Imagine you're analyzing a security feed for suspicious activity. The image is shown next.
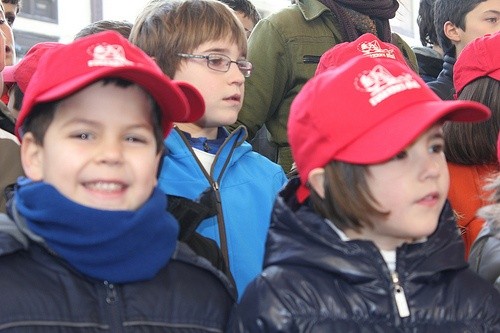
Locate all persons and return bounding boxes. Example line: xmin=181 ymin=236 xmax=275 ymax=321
xmin=127 ymin=0 xmax=289 ymax=304
xmin=224 ymin=55 xmax=500 ymax=333
xmin=218 ymin=0 xmax=281 ymax=164
xmin=0 ymin=0 xmax=134 ymax=212
xmin=409 ymin=0 xmax=444 ymax=84
xmin=425 ymin=0 xmax=500 ymax=101
xmin=446 ymin=28 xmax=500 ymax=260
xmin=0 ymin=30 xmax=241 ymax=333
xmin=226 ymin=0 xmax=419 ymax=170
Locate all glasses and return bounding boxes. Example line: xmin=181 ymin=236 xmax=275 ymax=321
xmin=176 ymin=53 xmax=252 ymax=78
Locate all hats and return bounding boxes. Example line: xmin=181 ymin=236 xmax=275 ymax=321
xmin=315 ymin=33 xmax=409 ymax=73
xmin=288 ymin=52 xmax=491 ymax=204
xmin=452 ymin=30 xmax=500 ymax=101
xmin=17 ymin=29 xmax=188 ymax=146
xmin=169 ymin=80 xmax=208 ymax=124
xmin=1 ymin=42 xmax=60 ymax=94
